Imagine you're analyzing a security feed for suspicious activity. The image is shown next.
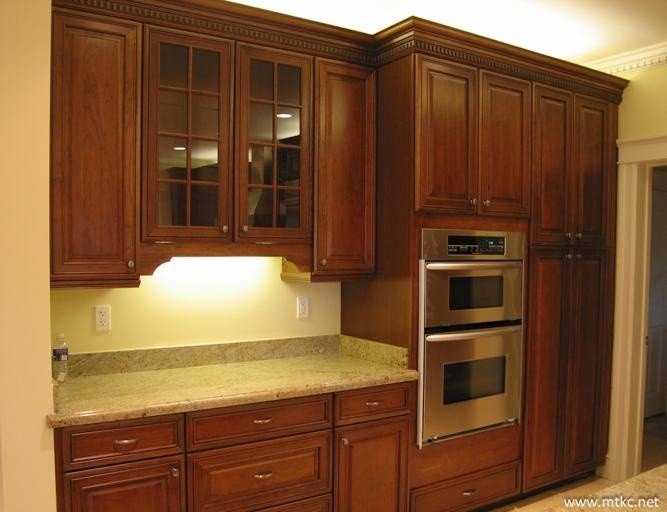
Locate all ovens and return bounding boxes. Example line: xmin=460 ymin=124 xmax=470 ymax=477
xmin=422 ymin=260 xmax=523 ymax=443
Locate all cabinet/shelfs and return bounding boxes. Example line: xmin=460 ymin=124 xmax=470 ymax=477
xmin=280 ymin=51 xmax=379 ymax=282
xmin=379 ymin=55 xmax=534 ymax=221
xmin=49 ymin=11 xmax=141 ymax=280
xmin=410 ymin=458 xmax=521 ymax=511
xmin=61 ymin=412 xmax=184 ymax=511
xmin=332 ymin=383 xmax=412 ymax=512
xmin=184 ymin=392 xmax=331 ymax=511
xmin=142 ymin=21 xmax=314 ymax=243
xmin=518 ymin=79 xmax=608 ymax=491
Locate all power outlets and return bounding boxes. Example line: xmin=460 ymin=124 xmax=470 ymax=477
xmin=94 ymin=305 xmax=111 ymax=332
xmin=296 ymin=296 xmax=309 ymax=319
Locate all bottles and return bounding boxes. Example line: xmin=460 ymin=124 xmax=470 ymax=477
xmin=52 ymin=331 xmax=70 ymax=386
